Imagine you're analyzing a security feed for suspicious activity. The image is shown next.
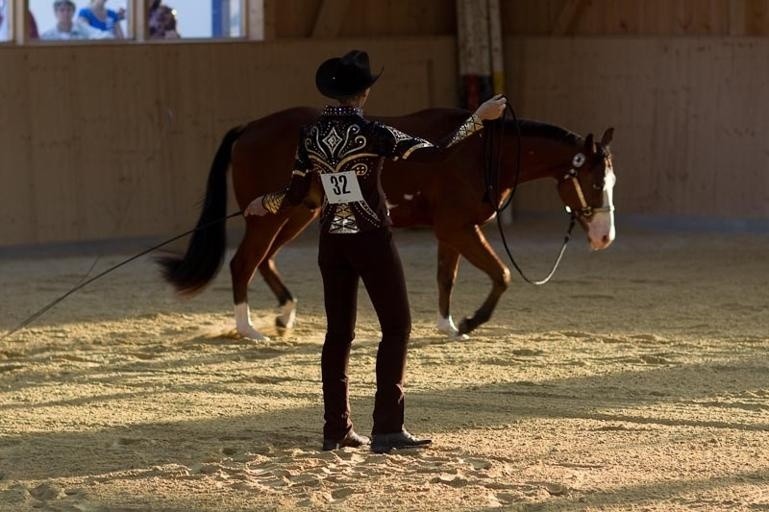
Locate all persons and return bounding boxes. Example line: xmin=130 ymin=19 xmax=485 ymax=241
xmin=243 ymin=50 xmax=507 ymax=453
xmin=75 ymin=0 xmax=126 ymax=40
xmin=0 ymin=1 xmax=39 ymax=43
xmin=39 ymin=1 xmax=87 ymax=42
xmin=146 ymin=1 xmax=180 ymax=41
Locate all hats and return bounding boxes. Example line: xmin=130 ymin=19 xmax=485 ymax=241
xmin=314 ymin=48 xmax=386 ymax=100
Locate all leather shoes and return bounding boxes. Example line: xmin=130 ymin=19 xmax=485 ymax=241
xmin=322 ymin=428 xmax=372 ymax=447
xmin=370 ymin=428 xmax=432 ymax=452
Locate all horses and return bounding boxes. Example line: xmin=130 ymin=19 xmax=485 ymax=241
xmin=156 ymin=106 xmax=617 ymax=341
